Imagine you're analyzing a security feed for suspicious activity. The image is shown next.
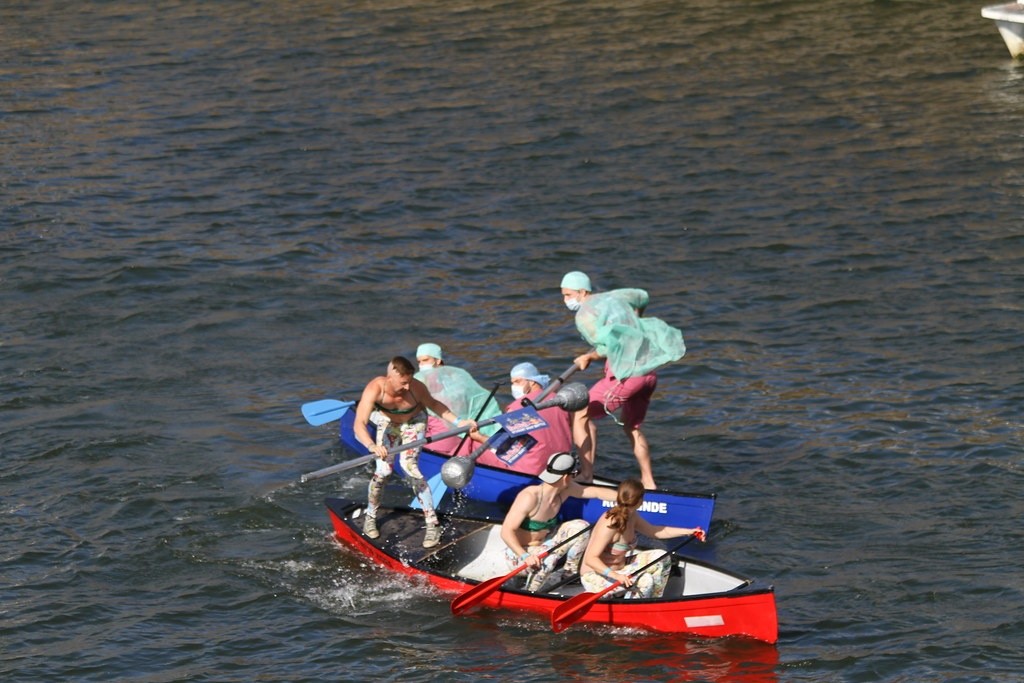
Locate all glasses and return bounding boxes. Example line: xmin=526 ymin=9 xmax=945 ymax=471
xmin=570 ymin=468 xmax=578 ymax=478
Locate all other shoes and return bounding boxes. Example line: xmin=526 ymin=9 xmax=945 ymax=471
xmin=560 ymin=574 xmax=582 ymax=587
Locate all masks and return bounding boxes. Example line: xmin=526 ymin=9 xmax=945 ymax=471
xmin=564 ymin=294 xmax=583 ymax=312
xmin=511 ymin=382 xmax=531 ymax=400
xmin=419 ymin=362 xmax=436 ymax=372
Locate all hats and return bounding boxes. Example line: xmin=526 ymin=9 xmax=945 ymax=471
xmin=538 ymin=452 xmax=576 ymax=483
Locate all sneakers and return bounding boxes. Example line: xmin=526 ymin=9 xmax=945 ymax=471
xmin=362 ymin=514 xmax=379 ymax=539
xmin=422 ymin=522 xmax=444 ymax=548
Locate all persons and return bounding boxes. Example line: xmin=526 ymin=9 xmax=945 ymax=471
xmin=560 ymin=271 xmax=686 ymax=489
xmin=501 ymin=451 xmax=618 ymax=594
xmin=413 ymin=343 xmax=503 ymax=456
xmin=579 ymin=478 xmax=705 ymax=599
xmin=353 ymin=356 xmax=477 ymax=548
xmin=449 ymin=362 xmax=574 ymax=479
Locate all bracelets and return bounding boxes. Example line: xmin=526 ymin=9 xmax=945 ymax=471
xmin=603 ymin=567 xmax=611 ymax=576
xmin=451 ymin=417 xmax=460 ymax=426
xmin=520 ymin=552 xmax=530 ymax=561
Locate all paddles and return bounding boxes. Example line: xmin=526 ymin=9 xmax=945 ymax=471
xmin=301 ymin=398 xmax=360 ymax=427
xmin=550 ymin=525 xmax=704 ymax=633
xmin=410 ymin=380 xmax=508 ymax=510
xmin=451 ymin=522 xmax=597 ymax=618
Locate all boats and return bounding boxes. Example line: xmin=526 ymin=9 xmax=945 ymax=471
xmin=323 ymin=496 xmax=779 ymax=645
xmin=982 ymin=0 xmax=1024 ymax=59
xmin=338 ymin=405 xmax=715 ymax=532
